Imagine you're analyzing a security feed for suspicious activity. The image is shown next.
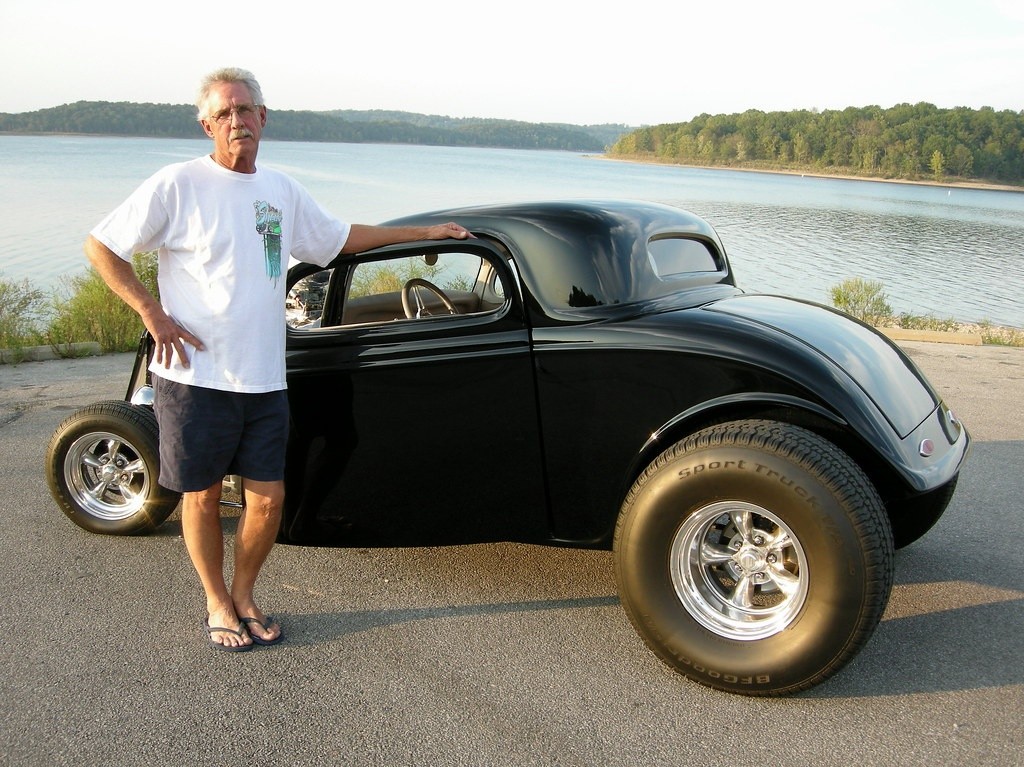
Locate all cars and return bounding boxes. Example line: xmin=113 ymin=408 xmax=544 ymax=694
xmin=47 ymin=201 xmax=970 ymax=697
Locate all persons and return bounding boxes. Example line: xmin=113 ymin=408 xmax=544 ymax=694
xmin=83 ymin=69 xmax=481 ymax=652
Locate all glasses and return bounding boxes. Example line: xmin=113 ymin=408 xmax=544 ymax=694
xmin=209 ymin=102 xmax=261 ymax=124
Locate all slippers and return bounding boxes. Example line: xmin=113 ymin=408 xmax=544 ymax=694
xmin=243 ymin=616 xmax=284 ymax=645
xmin=204 ymin=618 xmax=254 ymax=652
